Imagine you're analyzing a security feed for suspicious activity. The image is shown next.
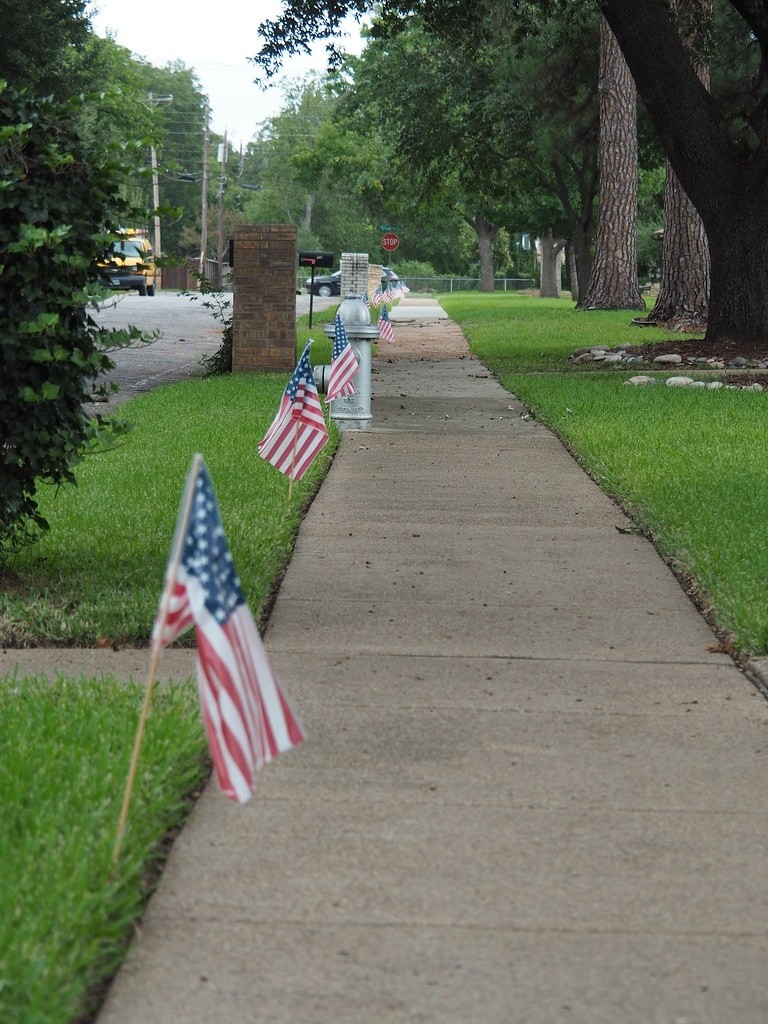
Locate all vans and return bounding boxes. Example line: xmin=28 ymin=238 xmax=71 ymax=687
xmin=93 ymin=228 xmax=160 ymax=297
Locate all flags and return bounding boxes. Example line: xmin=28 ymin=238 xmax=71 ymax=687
xmin=377 ymin=306 xmax=394 ymax=344
xmin=258 ymin=339 xmax=328 ymax=482
xmin=324 ymin=313 xmax=359 ymax=403
xmin=382 ymin=281 xmax=410 ymax=304
xmin=373 ymin=284 xmax=382 ymax=308
xmin=362 ymin=289 xmax=370 ymax=313
xmin=152 ymin=456 xmax=304 ymax=804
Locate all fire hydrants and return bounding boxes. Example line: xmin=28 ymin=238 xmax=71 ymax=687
xmin=312 ymin=292 xmax=378 ymax=434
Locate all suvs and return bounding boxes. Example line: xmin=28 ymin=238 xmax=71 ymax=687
xmin=305 ymin=265 xmax=400 ymax=296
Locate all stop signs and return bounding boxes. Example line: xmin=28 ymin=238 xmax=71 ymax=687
xmin=380 ymin=233 xmax=400 ymax=252
